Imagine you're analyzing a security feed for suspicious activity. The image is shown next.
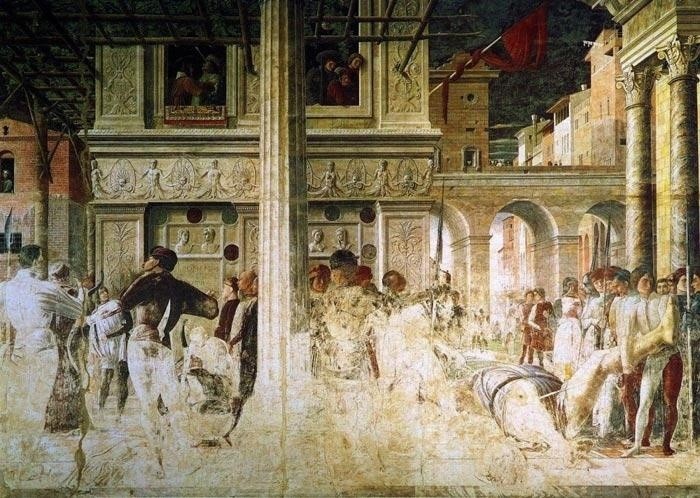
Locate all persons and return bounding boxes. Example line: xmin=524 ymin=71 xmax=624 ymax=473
xmin=228 ymin=178 xmax=261 ymax=190
xmin=90 ymin=160 xmax=109 ymax=200
xmin=310 ymin=160 xmax=353 ymax=197
xmin=419 ymin=157 xmax=434 ymax=196
xmin=134 ymin=158 xmax=171 ymax=200
xmin=110 ymin=177 xmax=133 ymax=194
xmin=342 ymin=174 xmax=373 ymax=188
xmin=392 ymin=174 xmax=423 ymax=194
xmin=164 ymin=177 xmax=201 ymax=192
xmin=194 ymin=159 xmax=234 ymax=199
xmin=0 ymin=169 xmax=13 ymax=193
xmin=367 ymin=158 xmax=397 ymax=198
xmin=166 ymin=48 xmax=363 ymax=110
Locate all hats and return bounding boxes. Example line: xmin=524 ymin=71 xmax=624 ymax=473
xmin=355 ymin=266 xmax=373 ymax=281
xmin=150 ymin=245 xmax=178 ymax=267
xmin=308 ymin=263 xmax=331 ymax=280
xmin=382 ymin=270 xmax=408 ymax=292
xmin=562 ymin=267 xmax=693 ymax=292
xmin=329 ymin=249 xmax=360 ymax=269
xmin=48 ymin=260 xmax=71 ymax=282
